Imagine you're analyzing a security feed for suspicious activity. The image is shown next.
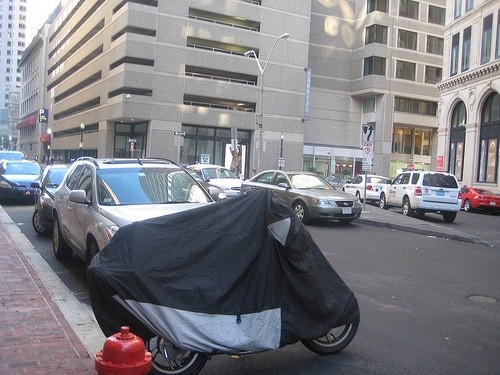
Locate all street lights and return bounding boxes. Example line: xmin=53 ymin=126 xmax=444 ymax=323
xmin=244 ymin=33 xmax=290 ymax=175
xmin=79 ymin=121 xmax=87 ymax=159
xmin=278 ymin=133 xmax=284 ymax=172
xmin=46 ymin=128 xmax=53 ymax=164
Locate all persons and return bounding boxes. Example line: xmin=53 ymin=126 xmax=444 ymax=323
xmin=25 ymin=154 xmax=54 ymax=166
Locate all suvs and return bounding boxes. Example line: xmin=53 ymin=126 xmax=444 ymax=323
xmin=51 ymin=155 xmax=219 ymax=274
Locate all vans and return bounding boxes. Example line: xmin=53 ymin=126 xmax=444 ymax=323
xmin=380 ymin=171 xmax=462 ymax=222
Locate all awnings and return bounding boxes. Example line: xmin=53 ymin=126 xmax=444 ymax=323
xmin=16 ymin=115 xmax=36 ymax=130
xmin=40 ymin=134 xmax=50 ymax=142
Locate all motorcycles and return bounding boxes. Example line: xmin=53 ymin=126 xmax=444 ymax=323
xmin=84 ymin=184 xmax=359 ymax=375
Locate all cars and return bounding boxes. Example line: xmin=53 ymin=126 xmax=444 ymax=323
xmin=0 ymin=159 xmax=43 ymax=206
xmin=458 ymin=186 xmax=500 ymax=212
xmin=242 ymin=170 xmax=363 ymax=224
xmin=341 ymin=174 xmax=391 ymax=203
xmin=30 ymin=164 xmax=75 ymax=235
xmin=1 ymin=151 xmax=26 ymax=162
xmin=187 ymin=164 xmax=244 ymax=201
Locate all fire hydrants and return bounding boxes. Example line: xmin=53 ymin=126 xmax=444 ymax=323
xmin=94 ymin=325 xmax=155 ymax=375
